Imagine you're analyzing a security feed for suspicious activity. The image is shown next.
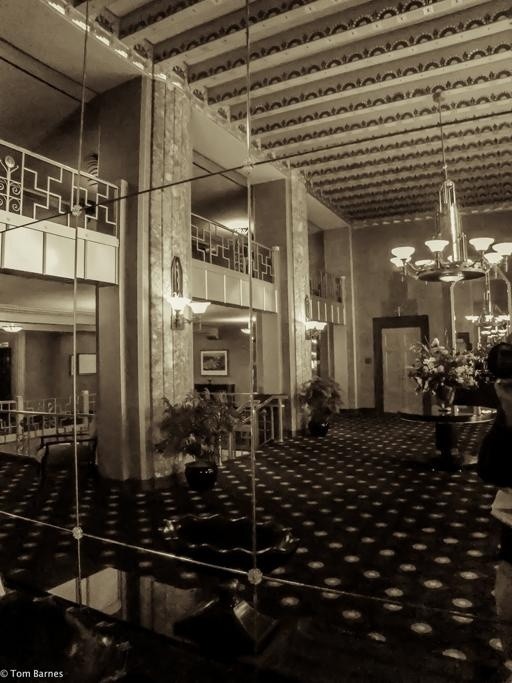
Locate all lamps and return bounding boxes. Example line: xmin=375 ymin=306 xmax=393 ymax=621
xmin=167 ymin=297 xmax=211 ymax=330
xmin=2 ymin=326 xmax=23 ymax=333
xmin=233 ymin=228 xmax=248 ymax=236
xmin=305 ymin=321 xmax=327 ymax=340
xmin=389 ymin=91 xmax=512 ymax=283
xmin=464 ymin=272 xmax=510 ymax=342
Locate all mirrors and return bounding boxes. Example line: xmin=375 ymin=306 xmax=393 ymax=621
xmin=449 ymin=264 xmax=511 ymax=355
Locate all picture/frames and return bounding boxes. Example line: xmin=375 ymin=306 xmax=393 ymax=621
xmin=77 ymin=352 xmax=97 ymax=375
xmin=200 ymin=349 xmax=230 ymax=377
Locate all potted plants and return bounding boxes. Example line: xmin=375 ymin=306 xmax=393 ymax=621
xmin=154 ymin=388 xmax=246 ymax=490
xmin=297 ymin=375 xmax=344 ymax=437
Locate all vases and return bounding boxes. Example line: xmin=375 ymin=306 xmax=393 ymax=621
xmin=430 ymin=384 xmax=456 ymax=412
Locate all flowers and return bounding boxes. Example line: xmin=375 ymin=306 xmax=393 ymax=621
xmin=405 ymin=328 xmax=483 ymax=395
